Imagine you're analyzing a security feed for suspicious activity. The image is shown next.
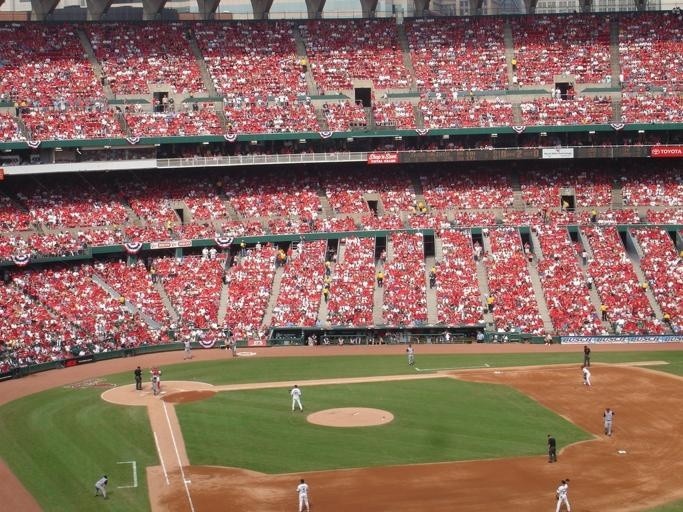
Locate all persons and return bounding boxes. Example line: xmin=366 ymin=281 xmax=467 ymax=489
xmin=0 ymin=11 xmax=682 ymax=375
xmin=135 ymin=367 xmax=142 ymax=390
xmin=290 ymin=385 xmax=303 ymax=412
xmin=94 ymin=475 xmax=109 ymax=499
xmin=602 ymin=408 xmax=616 ymax=436
xmin=296 ymin=478 xmax=310 ymax=512
xmin=546 ymin=434 xmax=557 ymax=463
xmin=555 ymin=478 xmax=571 ymax=512
xmin=149 ymin=367 xmax=162 ymax=391
xmin=580 ymin=365 xmax=593 ymax=391
